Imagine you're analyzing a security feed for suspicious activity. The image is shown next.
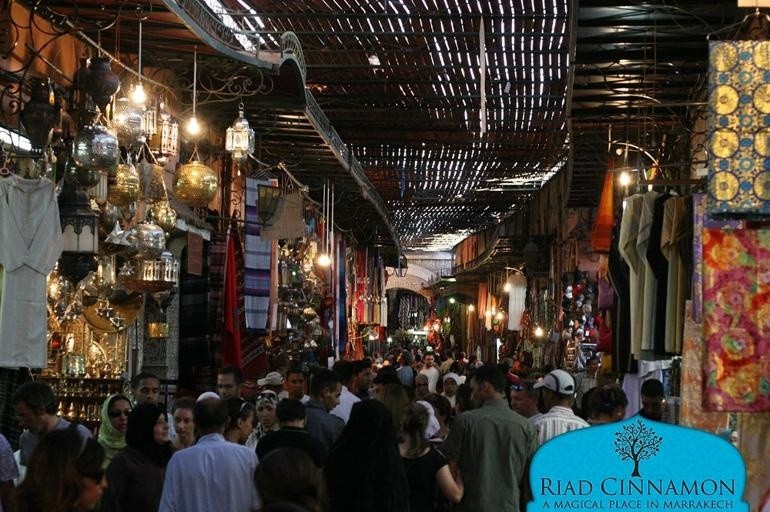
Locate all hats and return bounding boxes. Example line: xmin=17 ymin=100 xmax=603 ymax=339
xmin=257 ymin=372 xmax=285 ymax=386
xmin=533 ymin=369 xmax=575 ymax=395
xmin=416 ymin=400 xmax=441 ymax=439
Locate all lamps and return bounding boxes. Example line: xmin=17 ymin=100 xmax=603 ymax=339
xmin=185 ymin=45 xmax=203 ymax=135
xmin=128 ymin=18 xmax=148 ymax=105
xmin=225 ymin=97 xmax=257 ymax=178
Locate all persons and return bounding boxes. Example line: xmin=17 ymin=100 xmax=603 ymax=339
xmin=0 ymin=317 xmax=663 ymax=512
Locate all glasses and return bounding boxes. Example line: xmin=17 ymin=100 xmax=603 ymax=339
xmin=109 ymin=408 xmax=132 ymax=417
